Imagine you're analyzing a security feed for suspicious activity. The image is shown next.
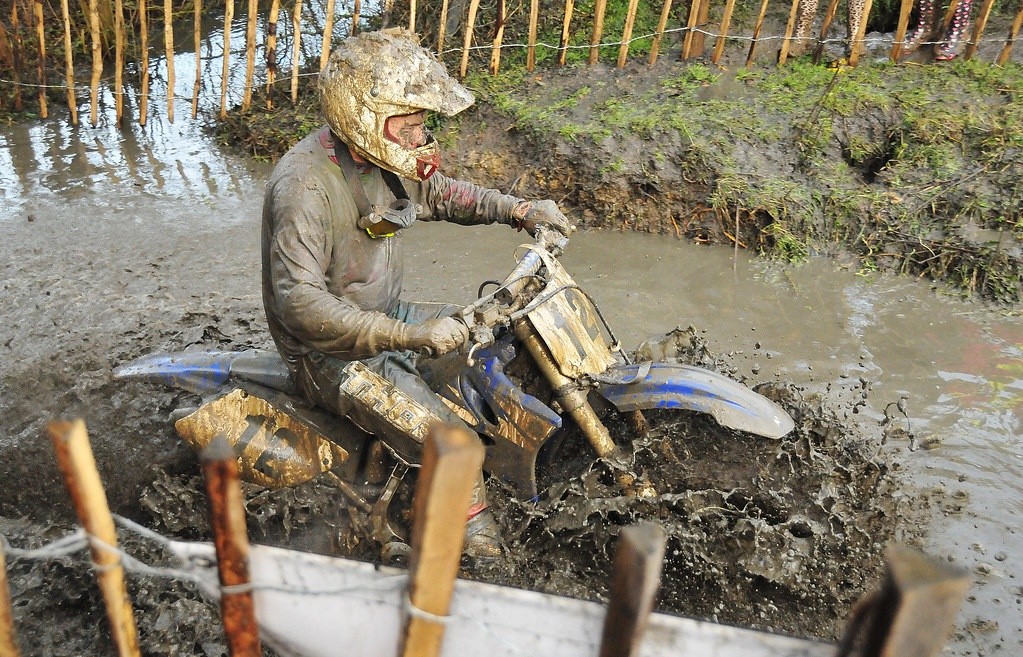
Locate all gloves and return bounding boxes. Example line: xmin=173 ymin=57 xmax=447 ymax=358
xmin=398 ymin=315 xmax=470 ymax=358
xmin=513 ymin=197 xmax=572 ymax=238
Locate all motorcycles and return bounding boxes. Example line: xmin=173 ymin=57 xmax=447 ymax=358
xmin=108 ymin=225 xmax=848 ymax=625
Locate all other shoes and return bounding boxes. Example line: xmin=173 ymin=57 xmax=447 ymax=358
xmin=461 ymin=509 xmax=502 ymax=560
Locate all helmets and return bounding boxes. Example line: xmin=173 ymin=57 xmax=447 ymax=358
xmin=316 ymin=31 xmax=475 ymax=183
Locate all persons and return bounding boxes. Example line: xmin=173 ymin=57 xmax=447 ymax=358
xmin=263 ymin=29 xmax=576 ymax=558
